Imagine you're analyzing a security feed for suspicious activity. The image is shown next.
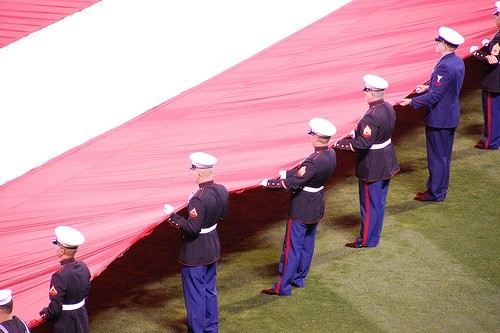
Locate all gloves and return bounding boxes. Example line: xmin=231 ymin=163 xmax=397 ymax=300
xmin=481 ymin=39 xmax=489 ymax=46
xmin=164 ymin=204 xmax=174 ymax=214
xmin=259 ymin=178 xmax=268 ymax=188
xmin=469 ymin=46 xmax=479 ymax=53
xmin=350 ymin=130 xmax=355 ymax=139
xmin=279 ymin=170 xmax=287 ymax=180
xmin=188 ymin=192 xmax=195 ymax=202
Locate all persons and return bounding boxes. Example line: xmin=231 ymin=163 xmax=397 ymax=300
xmin=0 ymin=289 xmax=32 ymax=333
xmin=469 ymin=0 xmax=500 ymax=150
xmin=398 ymin=26 xmax=466 ymax=202
xmin=163 ymin=152 xmax=230 ymax=333
xmin=333 ymin=74 xmax=401 ymax=248
xmin=258 ymin=118 xmax=337 ymax=296
xmin=38 ymin=226 xmax=92 ymax=333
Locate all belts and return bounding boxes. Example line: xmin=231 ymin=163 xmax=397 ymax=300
xmin=299 ymin=185 xmax=324 ymax=193
xmin=199 ymin=223 xmax=218 ymax=234
xmin=369 ymin=138 xmax=391 ymax=149
xmin=61 ymin=298 xmax=86 ymax=311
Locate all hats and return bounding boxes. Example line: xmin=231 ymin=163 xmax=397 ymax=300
xmin=306 ymin=117 xmax=336 ymax=138
xmin=0 ymin=289 xmax=12 ymax=306
xmin=493 ymin=1 xmax=500 ymax=15
xmin=361 ymin=74 xmax=389 ymax=91
xmin=52 ymin=226 xmax=85 ymax=249
xmin=188 ymin=152 xmax=217 ymax=170
xmin=435 ymin=26 xmax=466 ymax=47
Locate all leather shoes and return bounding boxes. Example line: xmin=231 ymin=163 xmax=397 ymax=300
xmin=414 ymin=191 xmax=428 ymax=201
xmin=261 ymin=288 xmax=279 ymax=295
xmin=344 ymin=240 xmax=368 ymax=248
xmin=474 ymin=139 xmax=498 ymax=150
